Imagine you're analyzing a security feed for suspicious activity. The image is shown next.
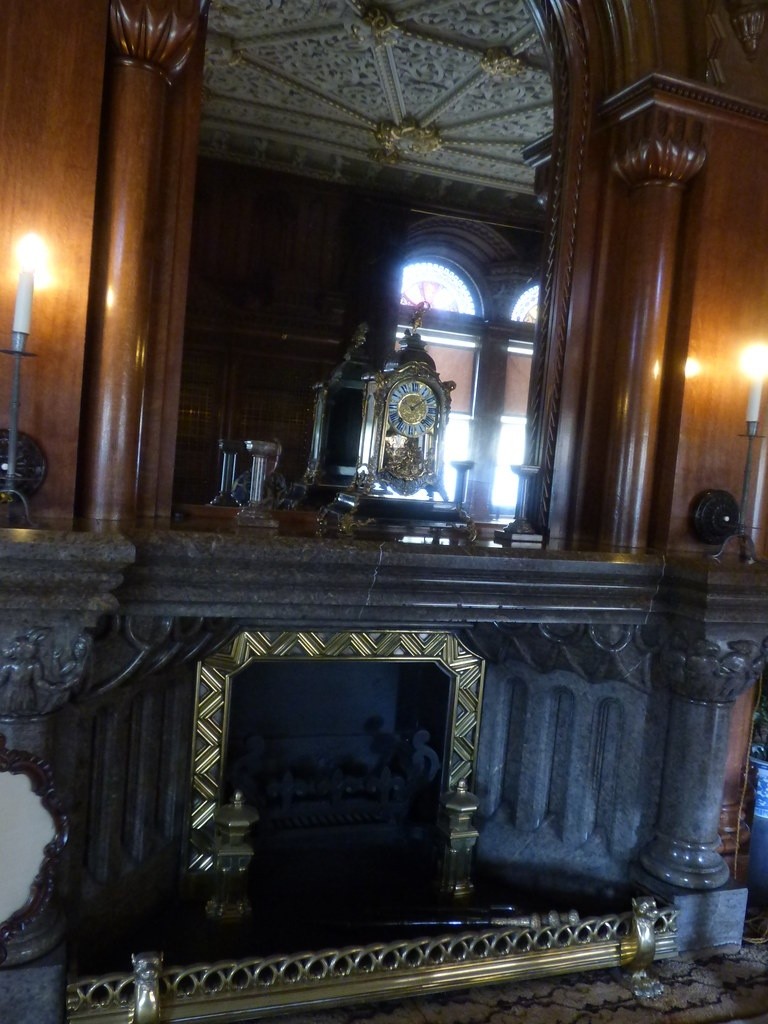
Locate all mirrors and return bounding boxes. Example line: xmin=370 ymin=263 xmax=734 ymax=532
xmin=172 ymin=0 xmax=565 ymax=531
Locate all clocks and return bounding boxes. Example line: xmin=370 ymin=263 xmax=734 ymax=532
xmin=276 ymin=322 xmax=379 ymax=511
xmin=315 ymin=332 xmax=478 ymax=545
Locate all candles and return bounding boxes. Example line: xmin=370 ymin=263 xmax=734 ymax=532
xmin=740 ymin=340 xmax=768 ymax=422
xmin=13 ymin=233 xmax=37 ymax=334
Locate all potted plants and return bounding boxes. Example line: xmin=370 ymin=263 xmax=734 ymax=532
xmin=744 ymin=695 xmax=768 ymax=818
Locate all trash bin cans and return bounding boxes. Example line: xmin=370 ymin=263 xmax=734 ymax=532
xmin=747 ymin=742 xmax=768 ymax=907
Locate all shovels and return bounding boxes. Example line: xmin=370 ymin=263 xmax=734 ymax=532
xmin=309 ymin=880 xmax=517 ymax=929
